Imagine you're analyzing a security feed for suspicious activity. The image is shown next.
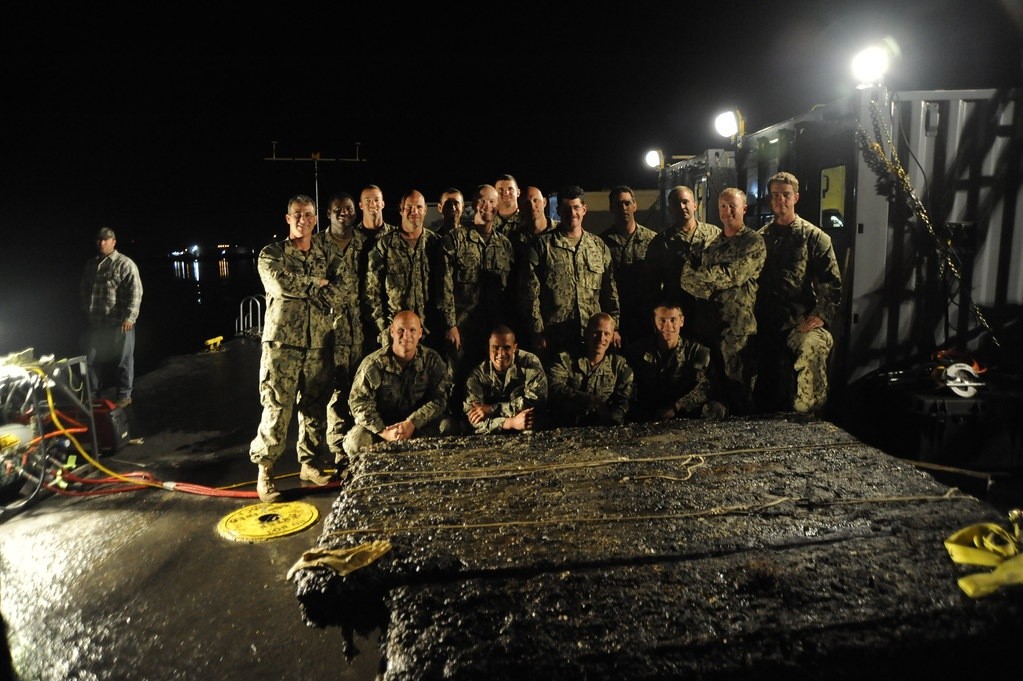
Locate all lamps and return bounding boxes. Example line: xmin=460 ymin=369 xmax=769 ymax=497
xmin=645 ymin=149 xmax=664 ymax=171
xmin=714 ymin=109 xmax=744 ymax=145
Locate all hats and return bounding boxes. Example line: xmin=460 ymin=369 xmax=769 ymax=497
xmin=97 ymin=227 xmax=115 ymax=239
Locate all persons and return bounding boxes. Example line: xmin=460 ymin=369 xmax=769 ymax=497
xmin=463 ymin=324 xmax=549 ymax=436
xmin=756 ymin=171 xmax=843 ymax=415
xmin=596 ymin=185 xmax=658 ymax=313
xmin=630 ymin=302 xmax=727 ymax=422
xmin=256 ymin=194 xmax=336 ymax=505
xmin=680 ymin=186 xmax=767 ymax=403
xmin=355 ymin=171 xmax=621 ymax=368
xmin=547 ymin=312 xmax=634 ymax=426
xmin=631 ymin=185 xmax=723 ymax=300
xmin=313 ymin=194 xmax=370 ymax=463
xmin=83 ymin=227 xmax=143 ymax=411
xmin=341 ymin=310 xmax=454 ymax=458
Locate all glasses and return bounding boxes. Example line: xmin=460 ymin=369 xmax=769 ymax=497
xmin=288 ymin=213 xmax=316 ymax=218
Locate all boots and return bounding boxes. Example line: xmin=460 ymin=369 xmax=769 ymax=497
xmin=257 ymin=463 xmax=282 ymax=502
xmin=301 ymin=463 xmax=333 ymax=486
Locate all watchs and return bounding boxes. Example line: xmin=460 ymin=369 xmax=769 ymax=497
xmin=491 ymin=403 xmax=502 ymax=416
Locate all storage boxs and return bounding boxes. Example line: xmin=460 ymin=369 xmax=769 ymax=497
xmin=901 ymin=371 xmax=1023 ymax=468
